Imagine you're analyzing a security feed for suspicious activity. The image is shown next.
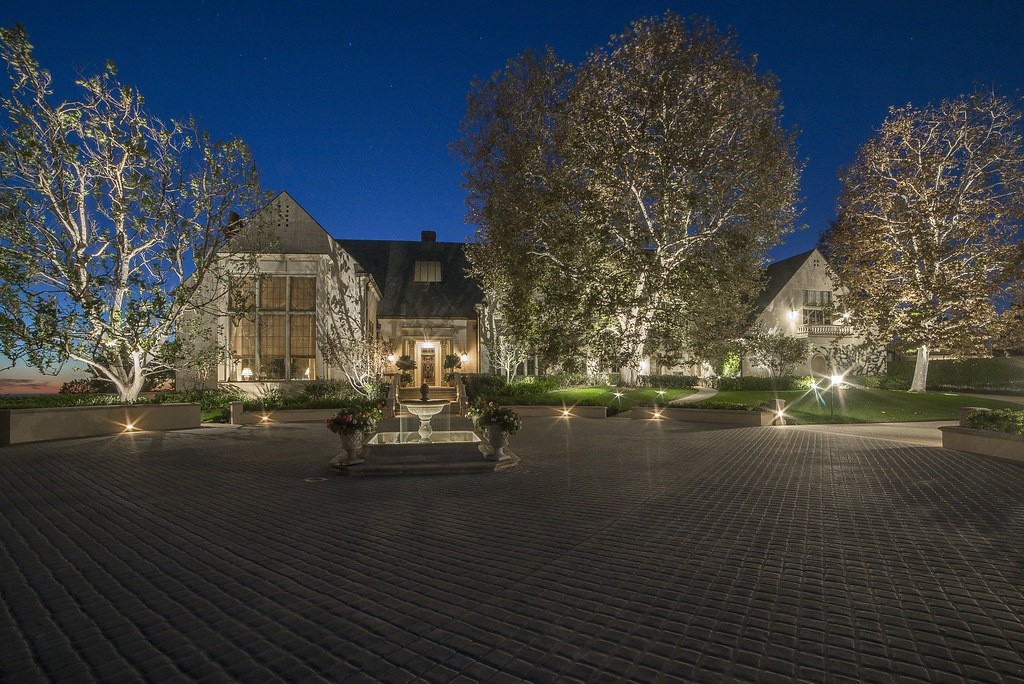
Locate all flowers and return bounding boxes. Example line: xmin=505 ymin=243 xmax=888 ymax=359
xmin=359 ymin=404 xmax=384 ymax=424
xmin=325 ymin=405 xmax=377 ymax=435
xmin=476 ymin=401 xmax=523 ymax=435
xmin=465 ymin=397 xmax=496 ymax=420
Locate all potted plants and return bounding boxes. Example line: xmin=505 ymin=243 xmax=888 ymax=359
xmin=396 ymin=354 xmax=418 ymax=387
xmin=442 ymin=353 xmax=462 ymax=387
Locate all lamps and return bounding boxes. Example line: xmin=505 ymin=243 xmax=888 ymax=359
xmin=241 ymin=368 xmax=253 ymax=381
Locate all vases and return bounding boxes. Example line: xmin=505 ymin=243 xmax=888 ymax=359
xmin=482 ymin=429 xmax=511 ymax=461
xmin=337 ymin=431 xmax=364 ymax=465
